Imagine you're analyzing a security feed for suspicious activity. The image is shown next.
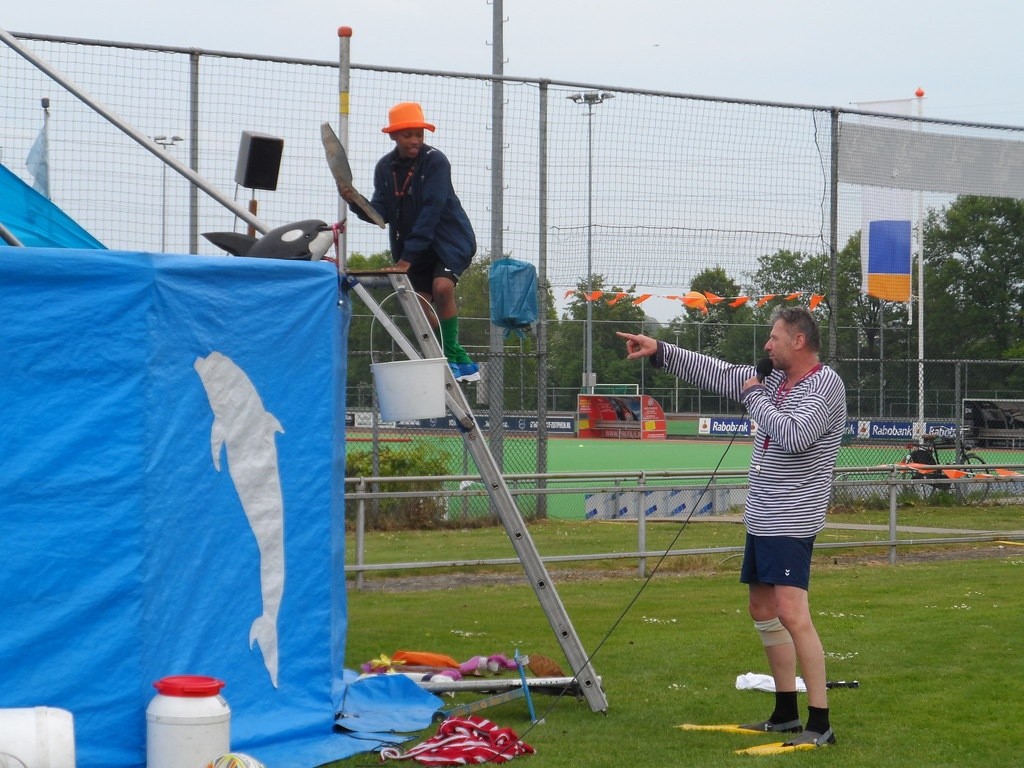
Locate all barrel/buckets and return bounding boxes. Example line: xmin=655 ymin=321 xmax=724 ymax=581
xmin=369 ymin=290 xmax=446 ymax=423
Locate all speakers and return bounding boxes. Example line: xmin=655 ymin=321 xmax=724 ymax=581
xmin=235 ymin=130 xmax=284 ymax=192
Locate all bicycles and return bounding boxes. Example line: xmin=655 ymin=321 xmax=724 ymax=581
xmin=902 ymin=426 xmax=991 ymax=508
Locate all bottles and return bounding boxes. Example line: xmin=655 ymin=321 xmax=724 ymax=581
xmin=146 ymin=677 xmax=231 ymax=768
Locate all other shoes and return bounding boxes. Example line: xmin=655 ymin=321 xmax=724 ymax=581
xmin=448 ymin=361 xmax=481 ymax=383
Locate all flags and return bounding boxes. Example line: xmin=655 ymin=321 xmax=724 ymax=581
xmin=860 ymin=103 xmax=912 ymax=303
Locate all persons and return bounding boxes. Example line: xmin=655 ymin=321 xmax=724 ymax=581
xmin=338 ymin=100 xmax=479 ymax=383
xmin=615 ymin=307 xmax=846 ymax=748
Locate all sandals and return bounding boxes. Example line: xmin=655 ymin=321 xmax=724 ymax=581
xmin=782 ymin=726 xmax=834 ymax=747
xmin=739 ymin=719 xmax=802 ymax=733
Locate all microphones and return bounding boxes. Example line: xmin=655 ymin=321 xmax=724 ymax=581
xmin=756 ymin=358 xmax=773 ymax=384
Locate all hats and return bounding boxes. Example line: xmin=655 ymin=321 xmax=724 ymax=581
xmin=382 ymin=102 xmax=435 ymax=131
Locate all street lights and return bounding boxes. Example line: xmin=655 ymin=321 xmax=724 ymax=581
xmin=566 ymin=90 xmax=616 ymax=393
xmin=149 ymin=133 xmax=184 ymax=253
